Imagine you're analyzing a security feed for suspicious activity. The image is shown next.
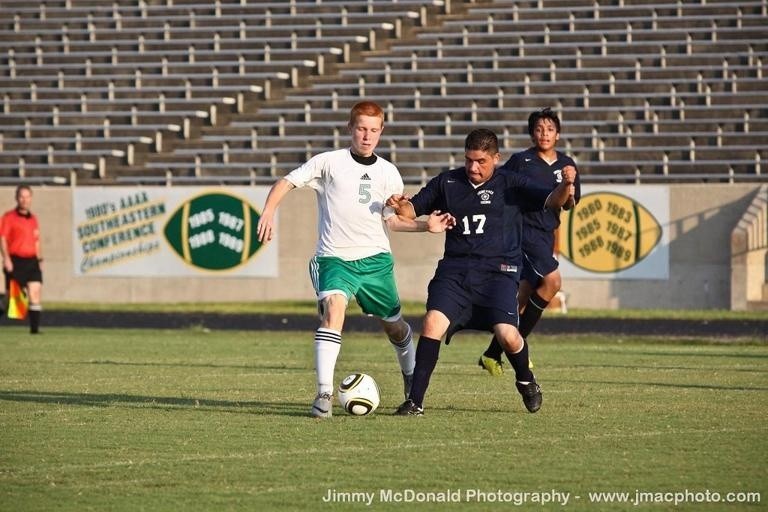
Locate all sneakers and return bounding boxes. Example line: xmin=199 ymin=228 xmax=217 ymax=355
xmin=515 ymin=371 xmax=542 ymax=413
xmin=402 ymin=370 xmax=414 ymax=400
xmin=478 ymin=354 xmax=505 ymax=376
xmin=392 ymin=398 xmax=424 ymax=416
xmin=311 ymin=391 xmax=334 ymax=417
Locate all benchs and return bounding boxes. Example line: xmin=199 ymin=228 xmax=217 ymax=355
xmin=0 ymin=0 xmax=768 ymax=186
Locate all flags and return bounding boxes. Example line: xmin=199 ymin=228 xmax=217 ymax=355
xmin=8 ymin=277 xmax=29 ymax=321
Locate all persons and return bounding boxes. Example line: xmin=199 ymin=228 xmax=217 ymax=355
xmin=0 ymin=183 xmax=45 ymax=333
xmin=387 ymin=128 xmax=577 ymax=417
xmin=256 ymin=101 xmax=456 ymax=419
xmin=477 ymin=108 xmax=581 ymax=379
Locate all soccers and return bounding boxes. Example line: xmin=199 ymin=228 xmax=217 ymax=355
xmin=339 ymin=373 xmax=380 ymax=415
